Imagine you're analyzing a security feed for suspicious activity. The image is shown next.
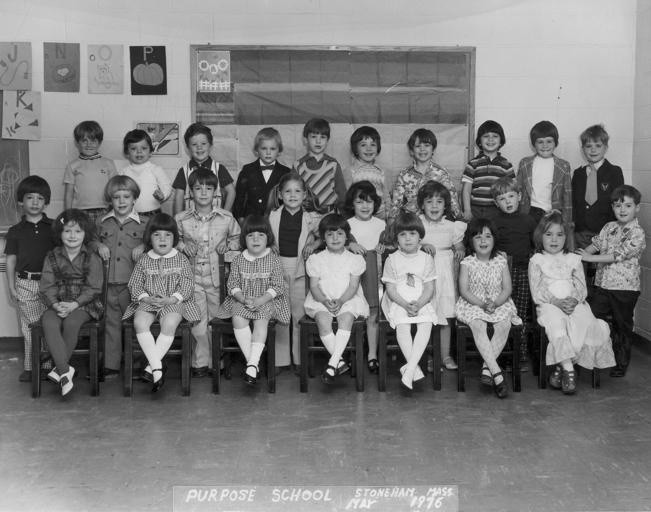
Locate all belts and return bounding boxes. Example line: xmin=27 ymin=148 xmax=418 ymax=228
xmin=18 ymin=271 xmax=42 ymax=280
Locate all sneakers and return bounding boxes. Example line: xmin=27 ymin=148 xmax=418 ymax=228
xmin=562 ymin=370 xmax=577 ymax=395
xmin=549 ymin=364 xmax=562 ymax=389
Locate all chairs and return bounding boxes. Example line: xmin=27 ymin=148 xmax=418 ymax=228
xmin=208 ymin=250 xmax=279 ymax=393
xmin=298 ymin=249 xmax=365 ymax=392
xmin=530 ymin=253 xmax=601 ymax=389
xmin=122 ymin=248 xmax=194 ymax=397
xmin=28 ymin=249 xmax=110 ymax=397
xmin=455 ymin=255 xmax=524 ymax=392
xmin=375 ymin=247 xmax=441 ymax=392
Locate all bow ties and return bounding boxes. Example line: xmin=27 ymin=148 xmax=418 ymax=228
xmin=258 ymin=164 xmax=275 ymax=171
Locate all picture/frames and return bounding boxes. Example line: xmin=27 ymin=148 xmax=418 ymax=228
xmin=133 ymin=120 xmax=184 ymax=158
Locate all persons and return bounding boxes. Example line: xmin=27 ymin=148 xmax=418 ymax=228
xmin=129 ymin=212 xmax=200 ymax=393
xmin=236 ymin=127 xmax=293 ymax=224
xmin=4 ymin=175 xmax=58 ymax=382
xmin=41 ymin=209 xmax=104 ymax=395
xmin=456 ymin=219 xmax=524 ymax=399
xmin=383 ymin=213 xmax=448 ymax=391
xmin=293 ymin=118 xmax=350 ymax=225
xmin=343 ymin=181 xmax=389 ymax=377
xmin=415 ymin=182 xmax=467 ymax=374
xmin=485 ymin=179 xmax=541 ymax=373
xmin=349 ymin=126 xmax=393 ymax=224
xmin=174 ymin=168 xmax=245 ymax=377
xmin=460 ymin=121 xmax=517 ymax=221
xmin=571 ymin=125 xmax=625 ymax=254
xmin=265 ymin=173 xmax=326 ymax=377
xmin=516 ymin=121 xmax=574 ymax=248
xmin=88 ymin=175 xmax=154 ymax=379
xmin=63 ymin=121 xmax=120 ymax=231
xmin=306 ymin=213 xmax=371 ymax=383
xmin=217 ymin=214 xmax=293 ymax=385
xmin=529 ymin=213 xmax=618 ymax=392
xmin=116 ymin=130 xmax=173 ymax=218
xmin=576 ymin=185 xmax=648 ymax=377
xmin=171 ymin=123 xmax=237 ymax=216
xmin=391 ymin=128 xmax=464 ymax=232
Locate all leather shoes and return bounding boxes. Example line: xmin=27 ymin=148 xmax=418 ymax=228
xmin=322 ymin=363 xmax=337 ymax=385
xmin=191 ymin=367 xmax=208 ymax=377
xmin=490 ymin=368 xmax=509 ymax=398
xmin=87 ymin=369 xmax=120 ymax=380
xmin=337 ymin=358 xmax=351 ymax=376
xmin=208 ymin=368 xmax=222 ymax=377
xmin=48 ymin=367 xmax=59 ymax=383
xmin=399 ymin=364 xmax=414 ymax=393
xmin=443 ymin=357 xmax=459 ymax=370
xmin=413 ymin=366 xmax=426 ymax=384
xmin=240 ymin=363 xmax=261 ymax=385
xmin=609 ymin=365 xmax=626 ymax=377
xmin=151 ymin=360 xmax=168 ymax=394
xmin=141 ymin=364 xmax=154 ymax=382
xmin=40 ymin=368 xmax=49 ymax=380
xmin=59 ymin=364 xmax=78 ymax=396
xmin=479 ymin=366 xmax=493 ymax=386
xmin=428 ymin=359 xmax=443 ymax=373
xmin=367 ymin=359 xmax=380 ymax=374
xmin=19 ymin=370 xmax=32 ymax=382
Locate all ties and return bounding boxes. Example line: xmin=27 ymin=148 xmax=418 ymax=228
xmin=584 ymin=161 xmax=597 ymax=205
